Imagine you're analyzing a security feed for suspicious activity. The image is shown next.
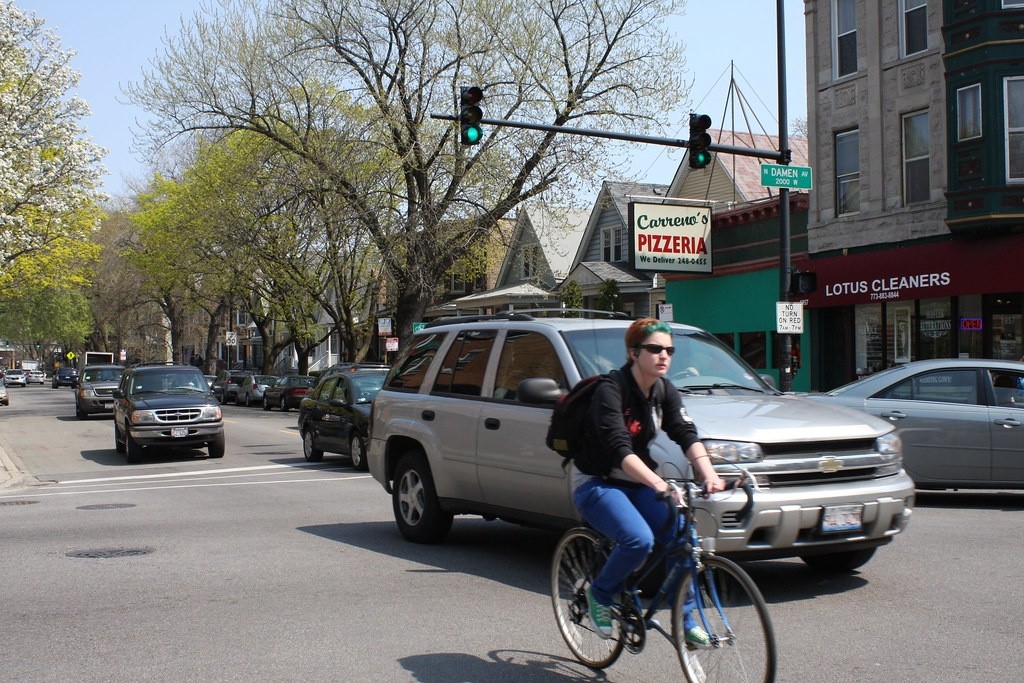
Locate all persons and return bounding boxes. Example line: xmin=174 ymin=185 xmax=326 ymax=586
xmin=994 ymin=376 xmax=1014 ymax=404
xmin=344 ymin=386 xmax=361 ymax=404
xmin=569 ymin=318 xmax=728 ymax=649
xmin=993 ymin=329 xmax=1002 ymax=353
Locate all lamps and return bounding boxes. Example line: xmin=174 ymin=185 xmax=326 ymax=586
xmin=842 ymin=247 xmax=849 ymax=256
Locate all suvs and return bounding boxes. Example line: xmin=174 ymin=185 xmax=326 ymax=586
xmin=209 ymin=370 xmax=258 ymax=405
xmin=73 ymin=362 xmax=127 ymax=421
xmin=366 ymin=306 xmax=917 ymax=596
xmin=51 ymin=366 xmax=77 ymax=389
xmin=112 ymin=360 xmax=227 ymax=463
xmin=306 ymin=361 xmax=391 ymax=395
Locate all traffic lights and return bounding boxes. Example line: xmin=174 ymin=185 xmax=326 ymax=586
xmin=6 ymin=341 xmax=10 ymax=348
xmin=459 ymin=85 xmax=484 ymax=145
xmin=792 ymin=271 xmax=818 ymax=295
xmin=37 ymin=342 xmax=40 ymax=347
xmin=688 ymin=113 xmax=712 ymax=169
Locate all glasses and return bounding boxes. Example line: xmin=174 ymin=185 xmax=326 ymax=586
xmin=634 ymin=344 xmax=676 ymax=357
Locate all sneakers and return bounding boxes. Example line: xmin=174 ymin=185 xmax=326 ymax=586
xmin=670 ymin=625 xmax=722 ymax=650
xmin=586 ymin=586 xmax=612 ymax=639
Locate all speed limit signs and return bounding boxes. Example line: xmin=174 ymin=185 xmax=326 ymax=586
xmin=225 ymin=331 xmax=237 ymax=346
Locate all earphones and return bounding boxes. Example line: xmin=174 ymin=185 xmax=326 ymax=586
xmin=634 ymin=350 xmax=639 ymax=357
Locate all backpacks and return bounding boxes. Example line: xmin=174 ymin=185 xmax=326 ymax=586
xmin=546 ymin=369 xmax=665 ymax=460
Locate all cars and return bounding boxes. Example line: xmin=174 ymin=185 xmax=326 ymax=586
xmin=235 ymin=374 xmax=280 ymax=407
xmin=297 ymin=369 xmax=390 ymax=470
xmin=203 ymin=374 xmax=218 ymax=388
xmin=262 ymin=376 xmax=318 ymax=412
xmin=786 ymin=357 xmax=1024 ymax=491
xmin=0 ymin=360 xmax=45 ymax=406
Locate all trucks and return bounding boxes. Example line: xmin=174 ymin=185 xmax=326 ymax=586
xmin=76 ymin=352 xmax=114 ymax=384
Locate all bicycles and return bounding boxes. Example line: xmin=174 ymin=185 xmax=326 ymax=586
xmin=550 ymin=454 xmax=777 ymax=683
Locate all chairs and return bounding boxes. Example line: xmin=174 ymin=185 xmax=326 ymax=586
xmin=993 ymin=375 xmax=1018 ymax=406
